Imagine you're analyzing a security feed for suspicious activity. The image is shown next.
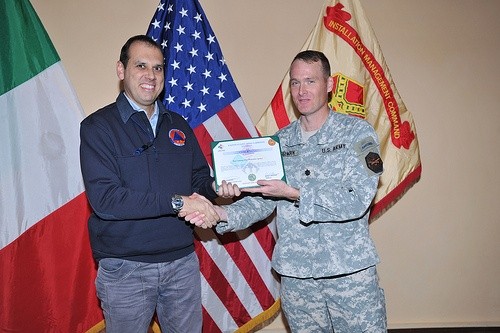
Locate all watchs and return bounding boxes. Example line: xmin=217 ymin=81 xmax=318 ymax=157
xmin=171 ymin=193 xmax=185 ymax=214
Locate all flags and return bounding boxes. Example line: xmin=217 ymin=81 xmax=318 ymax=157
xmin=256 ymin=0 xmax=423 ymax=221
xmin=1 ymin=0 xmax=106 ymax=333
xmin=146 ymin=0 xmax=283 ymax=333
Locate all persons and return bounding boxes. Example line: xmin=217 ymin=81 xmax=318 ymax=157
xmin=179 ymin=50 xmax=387 ymax=333
xmin=79 ymin=34 xmax=240 ymax=333
